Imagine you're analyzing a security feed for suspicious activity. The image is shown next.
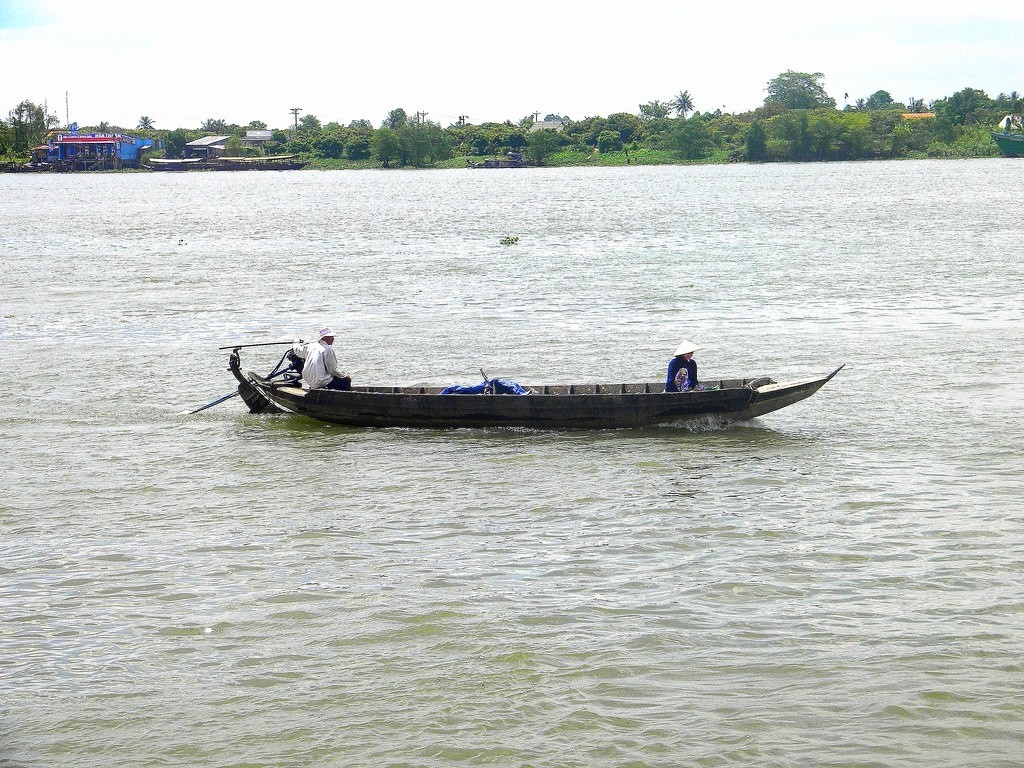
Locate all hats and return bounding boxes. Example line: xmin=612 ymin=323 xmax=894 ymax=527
xmin=673 ymin=340 xmax=705 ymax=356
xmin=318 ymin=327 xmax=338 ymax=343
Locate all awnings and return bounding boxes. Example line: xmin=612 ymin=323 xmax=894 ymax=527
xmin=30 ymin=144 xmax=59 ymax=162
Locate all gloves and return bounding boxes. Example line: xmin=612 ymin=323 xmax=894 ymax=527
xmin=293 ymin=336 xmax=300 ymax=343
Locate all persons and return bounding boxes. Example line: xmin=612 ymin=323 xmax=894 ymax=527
xmin=626 ymin=155 xmax=638 ymax=164
xmin=293 ymin=327 xmax=351 ymax=391
xmin=1006 ymin=118 xmax=1012 ymax=130
xmin=666 ymin=339 xmax=706 ymax=392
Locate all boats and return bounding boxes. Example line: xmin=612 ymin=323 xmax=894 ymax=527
xmin=989 ymin=131 xmax=1024 ymax=156
xmin=217 ymin=338 xmax=847 ymax=429
xmin=465 ymin=152 xmax=535 ymax=169
xmin=148 ymin=155 xmax=307 ymax=171
